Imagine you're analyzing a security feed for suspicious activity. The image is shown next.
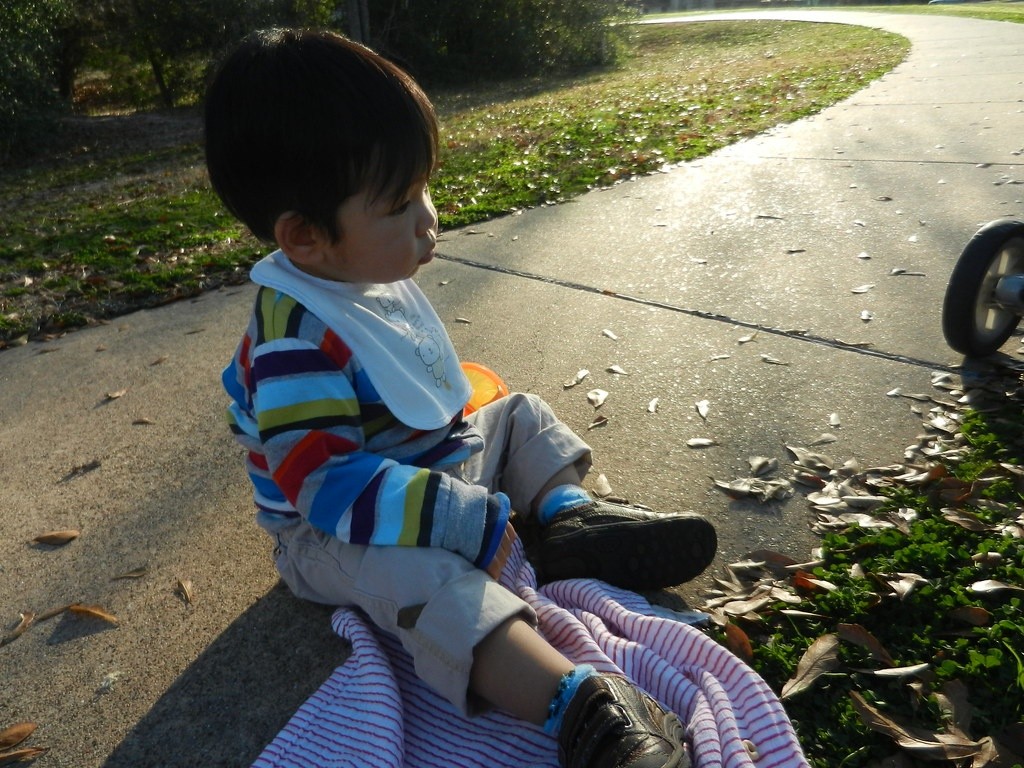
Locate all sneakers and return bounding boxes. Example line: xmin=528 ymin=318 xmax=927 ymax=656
xmin=538 ymin=495 xmax=718 ymax=592
xmin=556 ymin=676 xmax=692 ymax=768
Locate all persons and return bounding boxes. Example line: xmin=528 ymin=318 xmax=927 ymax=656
xmin=200 ymin=24 xmax=718 ymax=768
